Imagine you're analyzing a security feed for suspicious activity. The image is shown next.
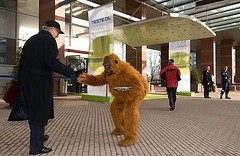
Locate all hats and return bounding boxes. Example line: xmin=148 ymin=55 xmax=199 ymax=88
xmin=45 ymin=20 xmax=65 ymax=34
xmin=168 ymin=58 xmax=174 ymax=63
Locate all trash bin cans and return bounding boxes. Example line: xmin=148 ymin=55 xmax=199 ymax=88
xmin=60 ymin=75 xmax=68 ymax=96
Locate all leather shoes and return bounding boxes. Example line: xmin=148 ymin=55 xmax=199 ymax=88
xmin=44 ymin=135 xmax=49 ymax=142
xmin=29 ymin=145 xmax=53 ymax=155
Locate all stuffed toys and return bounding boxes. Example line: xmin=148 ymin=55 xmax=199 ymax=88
xmin=76 ymin=53 xmax=147 ymax=147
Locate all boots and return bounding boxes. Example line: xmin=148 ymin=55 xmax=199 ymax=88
xmin=225 ymin=95 xmax=231 ymax=99
xmin=204 ymin=94 xmax=211 ymax=98
xmin=220 ymin=94 xmax=222 ymax=99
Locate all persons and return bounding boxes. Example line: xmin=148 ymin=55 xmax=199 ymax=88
xmin=160 ymin=59 xmax=181 ymax=110
xmin=19 ymin=20 xmax=78 ymax=155
xmin=202 ymin=66 xmax=216 ymax=98
xmin=220 ymin=65 xmax=231 ymax=99
